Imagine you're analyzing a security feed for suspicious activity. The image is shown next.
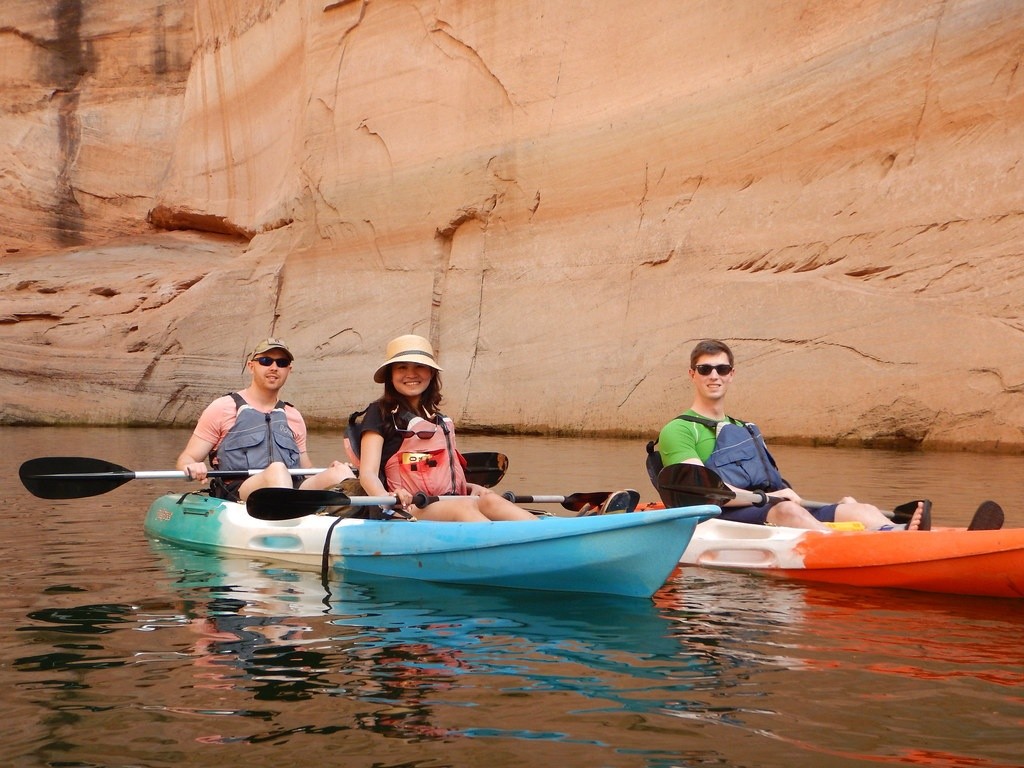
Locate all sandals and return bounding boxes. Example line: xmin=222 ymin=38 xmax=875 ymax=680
xmin=967 ymin=500 xmax=1005 ymax=530
xmin=904 ymin=498 xmax=932 ymax=531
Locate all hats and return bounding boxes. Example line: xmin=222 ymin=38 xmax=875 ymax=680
xmin=373 ymin=334 xmax=444 ymax=384
xmin=252 ymin=337 xmax=294 ymax=363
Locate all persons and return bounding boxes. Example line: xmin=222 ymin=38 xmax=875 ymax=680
xmin=172 ymin=338 xmax=363 ymax=516
xmin=358 ymin=335 xmax=642 ymax=521
xmin=659 ymin=340 xmax=1006 ymax=534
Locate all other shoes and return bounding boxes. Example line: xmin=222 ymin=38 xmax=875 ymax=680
xmin=597 ymin=488 xmax=631 ymax=515
xmin=624 ymin=489 xmax=640 ymax=512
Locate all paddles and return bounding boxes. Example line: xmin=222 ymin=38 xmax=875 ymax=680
xmin=657 ymin=463 xmax=933 ymax=524
xmin=246 ymin=485 xmax=641 ymax=521
xmin=18 ymin=449 xmax=511 ymax=501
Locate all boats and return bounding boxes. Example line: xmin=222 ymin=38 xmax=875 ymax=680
xmin=141 ymin=490 xmax=722 ymax=600
xmin=590 ymin=499 xmax=1023 ymax=601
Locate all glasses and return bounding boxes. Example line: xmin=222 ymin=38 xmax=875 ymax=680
xmin=252 ymin=356 xmax=290 ymax=369
xmin=391 ymin=406 xmax=438 ymax=440
xmin=693 ymin=363 xmax=733 ymax=376
